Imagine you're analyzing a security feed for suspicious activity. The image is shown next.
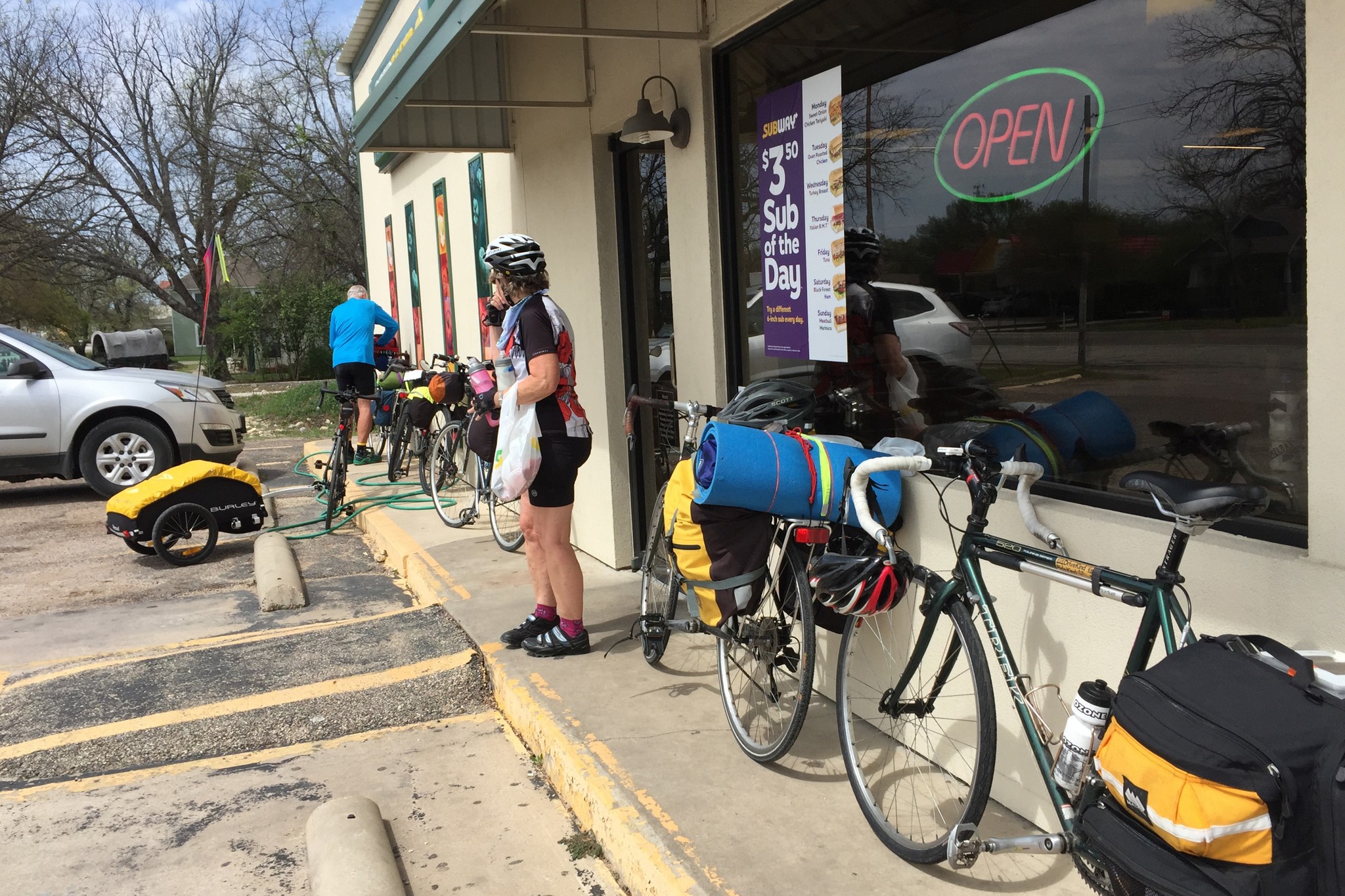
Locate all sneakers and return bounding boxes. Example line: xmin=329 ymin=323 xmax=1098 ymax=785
xmin=521 ymin=625 xmax=590 ymax=657
xmin=353 ymin=451 xmax=382 ymax=465
xmin=346 ymin=453 xmax=354 ymax=464
xmin=500 ymin=613 xmax=560 ymax=644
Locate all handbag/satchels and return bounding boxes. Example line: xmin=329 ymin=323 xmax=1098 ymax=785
xmin=490 ymin=382 xmax=542 ymax=503
xmin=373 ymin=334 xmax=400 ymax=371
xmin=465 ymin=407 xmax=501 ymax=463
xmin=885 ymin=356 xmax=928 ymax=440
xmin=405 ymin=386 xmax=434 ymax=429
xmin=779 ymin=519 xmax=878 ymax=634
xmin=446 ymin=403 xmax=467 ymax=432
xmin=1082 ymin=634 xmax=1345 ymax=896
xmin=664 ymin=452 xmax=773 ymax=631
xmin=370 ymin=389 xmax=395 ymax=426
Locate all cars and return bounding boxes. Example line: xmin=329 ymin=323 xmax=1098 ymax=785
xmin=650 ymin=281 xmax=979 ymax=413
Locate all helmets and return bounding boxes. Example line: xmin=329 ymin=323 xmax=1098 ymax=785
xmin=808 ymin=552 xmax=914 ymax=618
xmin=907 ymin=365 xmax=1007 ymax=426
xmin=719 ymin=377 xmax=818 ymax=434
xmin=844 ymin=226 xmax=880 ymax=259
xmin=482 ymin=234 xmax=547 ymax=276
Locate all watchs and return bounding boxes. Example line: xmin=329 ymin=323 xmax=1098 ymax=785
xmin=498 ymin=391 xmax=503 ymax=406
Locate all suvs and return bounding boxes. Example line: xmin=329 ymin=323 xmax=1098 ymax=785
xmin=1 ymin=322 xmax=249 ymax=499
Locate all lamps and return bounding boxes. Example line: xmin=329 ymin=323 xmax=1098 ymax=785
xmin=618 ymin=76 xmax=691 ymax=151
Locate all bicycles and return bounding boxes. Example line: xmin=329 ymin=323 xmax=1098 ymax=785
xmin=1074 ymin=418 xmax=1307 ymax=516
xmin=315 ymin=381 xmax=384 ymax=530
xmin=622 ymin=385 xmax=827 ymax=766
xmin=835 ymin=440 xmax=1345 ymax=896
xmin=378 ymin=349 xmax=528 ymax=551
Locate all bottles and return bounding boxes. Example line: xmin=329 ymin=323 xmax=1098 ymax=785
xmin=1268 ymin=370 xmax=1302 ymax=473
xmin=1054 ymin=679 xmax=1116 ymax=793
xmin=494 ymin=350 xmax=516 ymax=392
xmin=467 ymin=356 xmax=502 ymax=427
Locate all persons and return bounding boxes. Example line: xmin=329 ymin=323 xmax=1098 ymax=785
xmin=812 ymin=227 xmax=906 ymax=449
xmin=482 ymin=234 xmax=592 ymax=657
xmin=329 ymin=285 xmax=400 ymax=465
